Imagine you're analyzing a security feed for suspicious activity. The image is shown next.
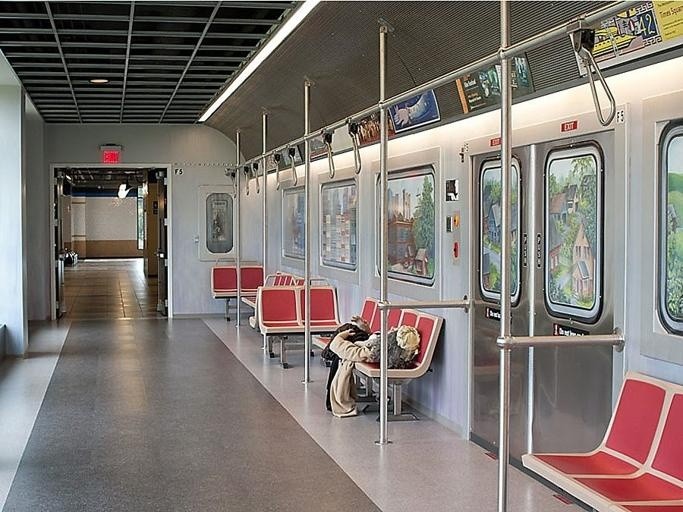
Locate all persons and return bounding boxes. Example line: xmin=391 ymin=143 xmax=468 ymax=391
xmin=321 ymin=315 xmax=420 ymax=418
xmin=393 ymin=91 xmax=433 ymax=126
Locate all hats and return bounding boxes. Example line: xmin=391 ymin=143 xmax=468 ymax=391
xmin=396 ymin=325 xmax=421 ymax=350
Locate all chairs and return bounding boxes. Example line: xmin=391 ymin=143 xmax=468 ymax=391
xmin=258 ymin=287 xmax=306 ymax=368
xmin=312 ymin=297 xmax=378 ymax=356
xmin=606 ymin=501 xmax=683 ymax=512
xmin=563 ymin=387 xmax=683 ymax=511
xmin=335 ymin=303 xmax=392 ymax=377
xmin=356 ymin=309 xmax=420 ymax=413
xmin=353 ymin=312 xmax=444 ymax=422
xmin=298 ymin=287 xmax=341 ymax=364
xmin=240 ymin=265 xmax=265 ymax=296
xmin=521 ymin=370 xmax=672 ymax=483
xmin=347 ymin=305 xmax=402 ymax=399
xmin=211 ymin=267 xmax=237 ymax=321
